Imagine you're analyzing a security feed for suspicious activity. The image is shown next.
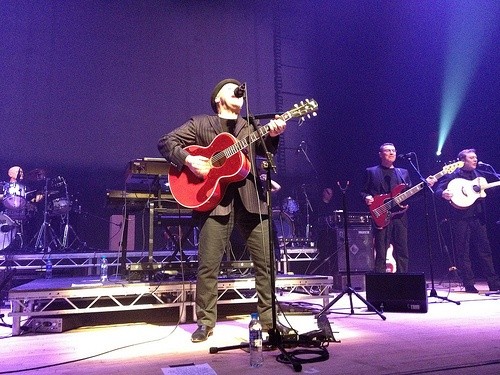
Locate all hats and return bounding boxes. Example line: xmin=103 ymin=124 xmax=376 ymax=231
xmin=210 ymin=79 xmax=243 ymax=113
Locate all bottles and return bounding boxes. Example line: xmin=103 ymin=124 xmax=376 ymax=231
xmin=46 ymin=257 xmax=52 ymax=279
xmin=248 ymin=313 xmax=264 ymax=368
xmin=100 ymin=255 xmax=108 ymax=282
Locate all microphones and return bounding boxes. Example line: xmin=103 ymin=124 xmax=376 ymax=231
xmin=397 ymin=152 xmax=410 ymax=160
xmin=15 ymin=168 xmax=21 ymax=180
xmin=478 ymin=161 xmax=490 ymax=167
xmin=234 ymin=83 xmax=245 ymax=97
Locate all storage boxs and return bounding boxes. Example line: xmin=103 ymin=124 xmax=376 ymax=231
xmin=110 ymin=215 xmax=135 ymax=251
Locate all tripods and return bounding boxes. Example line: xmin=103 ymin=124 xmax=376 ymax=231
xmin=407 ymin=158 xmax=460 ymax=306
xmin=12 ymin=176 xmax=89 ymax=253
xmin=314 ymin=191 xmax=386 ymax=321
xmin=209 ymin=85 xmax=321 ymax=372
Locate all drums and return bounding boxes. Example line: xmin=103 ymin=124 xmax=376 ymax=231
xmin=0 ymin=212 xmax=30 ymax=253
xmin=53 ymin=198 xmax=73 ymax=215
xmin=3 ymin=201 xmax=35 ymax=219
xmin=2 ymin=182 xmax=26 ymax=209
xmin=271 ymin=209 xmax=295 ymax=247
xmin=280 ymin=196 xmax=300 ymax=214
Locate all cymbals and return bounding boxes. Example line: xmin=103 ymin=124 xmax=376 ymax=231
xmin=36 ymin=190 xmax=59 ymax=195
xmin=26 ymin=168 xmax=48 ymax=181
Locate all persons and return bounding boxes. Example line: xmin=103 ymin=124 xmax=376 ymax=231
xmin=315 ymin=186 xmax=343 ymax=215
xmin=360 ymin=142 xmax=437 ymax=274
xmin=434 ymin=148 xmax=500 ymax=293
xmin=0 ymin=166 xmax=44 ymax=203
xmin=158 ymin=79 xmax=288 ymax=342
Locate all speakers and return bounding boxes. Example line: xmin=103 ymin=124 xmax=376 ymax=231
xmin=319 ymin=224 xmax=374 ymax=292
xmin=109 ymin=214 xmax=135 ymax=251
xmin=365 ymin=271 xmax=428 ymax=312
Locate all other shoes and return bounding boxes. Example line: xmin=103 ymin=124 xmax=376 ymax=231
xmin=191 ymin=325 xmax=214 ymax=341
xmin=268 ymin=324 xmax=295 ymax=334
xmin=465 ymin=286 xmax=479 ymax=293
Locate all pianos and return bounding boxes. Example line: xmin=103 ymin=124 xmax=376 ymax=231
xmin=104 ymin=157 xmax=199 ymax=281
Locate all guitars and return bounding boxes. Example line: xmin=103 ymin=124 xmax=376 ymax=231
xmin=446 ymin=176 xmax=500 ymax=209
xmin=369 ymin=157 xmax=465 ymax=229
xmin=168 ymin=98 xmax=320 ymax=211
xmin=374 ymin=243 xmax=397 ymax=273
